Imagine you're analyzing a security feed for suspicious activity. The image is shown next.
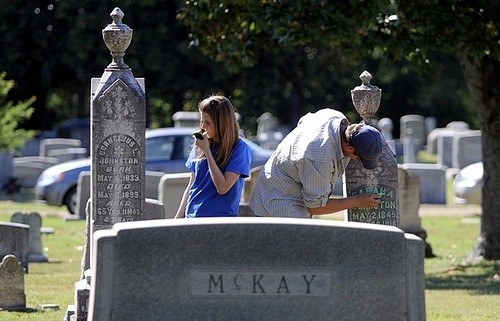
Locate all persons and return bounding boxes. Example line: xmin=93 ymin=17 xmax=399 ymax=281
xmin=246 ymin=108 xmax=383 ymax=219
xmin=174 ymin=95 xmax=253 ymax=218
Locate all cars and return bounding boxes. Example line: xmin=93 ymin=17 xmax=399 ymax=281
xmin=33 ymin=125 xmax=274 ymax=217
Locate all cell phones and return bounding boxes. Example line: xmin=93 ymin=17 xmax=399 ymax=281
xmin=193 ymin=133 xmax=204 ymax=140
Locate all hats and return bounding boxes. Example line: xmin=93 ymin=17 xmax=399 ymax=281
xmin=351 ymin=125 xmax=384 ymax=170
